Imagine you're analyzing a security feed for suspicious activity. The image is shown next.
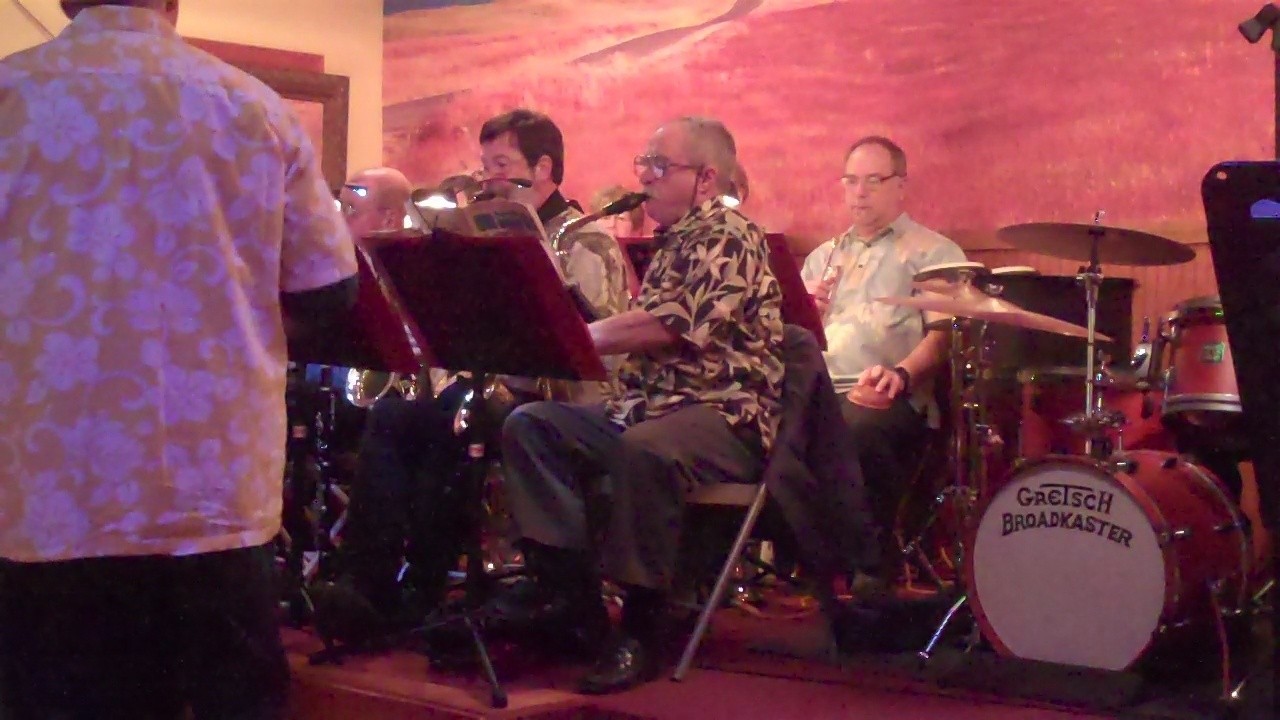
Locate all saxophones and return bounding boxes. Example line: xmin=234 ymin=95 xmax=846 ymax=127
xmin=339 ymin=189 xmax=502 ymax=416
xmin=452 ymin=188 xmax=651 ymax=534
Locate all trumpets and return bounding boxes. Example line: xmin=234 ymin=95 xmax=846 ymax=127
xmin=796 ymin=236 xmax=844 ymax=334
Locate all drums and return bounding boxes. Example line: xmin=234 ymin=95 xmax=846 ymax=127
xmin=961 ymin=445 xmax=1252 ymax=679
xmin=1161 ymin=290 xmax=1259 ymax=460
xmin=964 ymin=268 xmax=1144 ymax=386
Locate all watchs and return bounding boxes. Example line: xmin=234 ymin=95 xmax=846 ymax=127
xmin=893 ymin=367 xmax=910 ymax=394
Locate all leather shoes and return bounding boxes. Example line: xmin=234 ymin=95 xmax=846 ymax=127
xmin=577 ymin=635 xmax=662 ymax=694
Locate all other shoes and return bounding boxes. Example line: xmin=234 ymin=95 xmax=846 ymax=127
xmin=314 ymin=585 xmax=400 ymax=650
xmin=851 ymin=570 xmax=896 ymax=607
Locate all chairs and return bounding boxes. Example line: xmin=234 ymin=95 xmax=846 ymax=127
xmin=666 ymin=324 xmax=848 ymax=681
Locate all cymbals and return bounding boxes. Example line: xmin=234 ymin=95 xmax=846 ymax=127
xmin=870 ymin=282 xmax=1120 ymax=348
xmin=993 ymin=221 xmax=1198 ymax=266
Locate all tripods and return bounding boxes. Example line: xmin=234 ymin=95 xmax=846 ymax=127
xmin=306 ymin=369 xmax=548 ymax=713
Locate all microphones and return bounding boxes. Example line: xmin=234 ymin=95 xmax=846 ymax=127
xmin=472 ymin=175 xmax=535 ymax=192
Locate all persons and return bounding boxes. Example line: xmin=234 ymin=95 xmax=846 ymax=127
xmin=287 ymin=109 xmax=750 ymax=694
xmin=0 ymin=0 xmax=359 ymax=720
xmin=799 ymin=135 xmax=971 ymax=595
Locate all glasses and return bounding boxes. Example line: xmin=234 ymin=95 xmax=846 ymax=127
xmin=336 ymin=199 xmax=391 ymax=217
xmin=633 ymin=153 xmax=704 ymax=179
xmin=837 ymin=172 xmax=897 ymax=190
